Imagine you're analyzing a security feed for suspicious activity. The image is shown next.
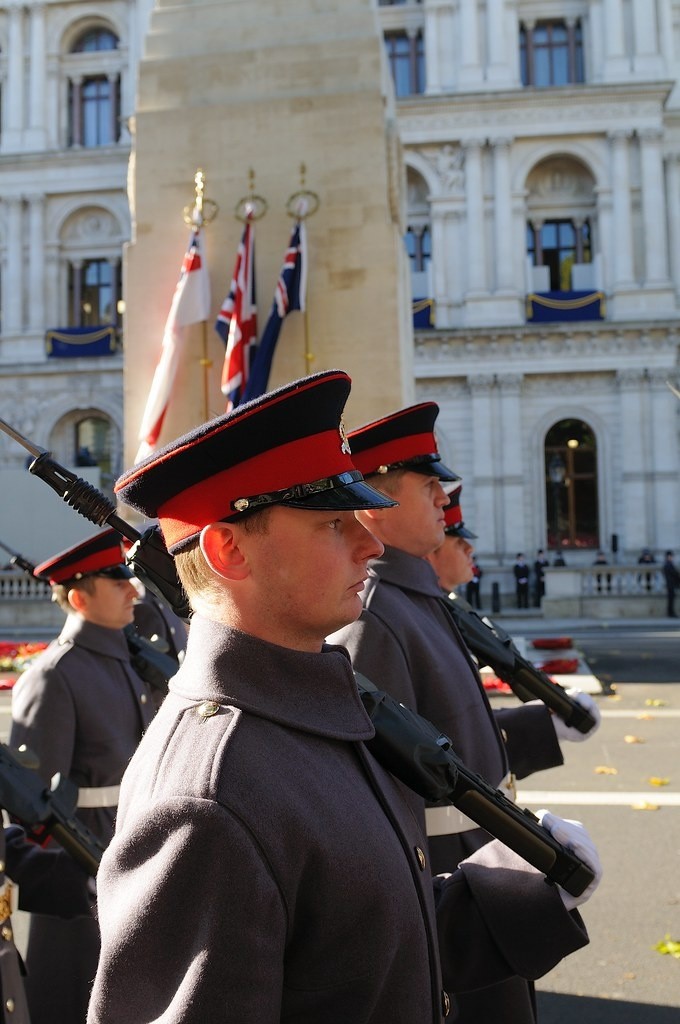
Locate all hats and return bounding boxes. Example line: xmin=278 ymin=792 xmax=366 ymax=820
xmin=112 ymin=369 xmax=401 ymax=556
xmin=347 ymin=400 xmax=463 ymax=482
xmin=32 ymin=527 xmax=136 ymax=585
xmin=442 ymin=482 xmax=478 ymax=540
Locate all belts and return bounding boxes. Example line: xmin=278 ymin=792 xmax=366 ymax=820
xmin=423 ymin=771 xmax=517 ymax=837
xmin=77 ymin=785 xmax=123 ymax=809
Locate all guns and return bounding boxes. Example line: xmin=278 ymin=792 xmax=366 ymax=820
xmin=0 ymin=418 xmax=597 ymax=900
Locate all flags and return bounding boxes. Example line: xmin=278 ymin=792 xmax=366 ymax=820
xmin=133 ymin=210 xmax=306 ymax=468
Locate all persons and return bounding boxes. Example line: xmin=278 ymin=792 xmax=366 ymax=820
xmin=638 ymin=547 xmax=680 ymax=618
xmin=594 ymin=551 xmax=611 ymax=592
xmin=0 ymin=370 xmax=601 ymax=1024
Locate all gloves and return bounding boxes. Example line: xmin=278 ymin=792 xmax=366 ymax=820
xmin=535 ymin=807 xmax=603 ymax=911
xmin=550 ymin=689 xmax=603 ymax=744
